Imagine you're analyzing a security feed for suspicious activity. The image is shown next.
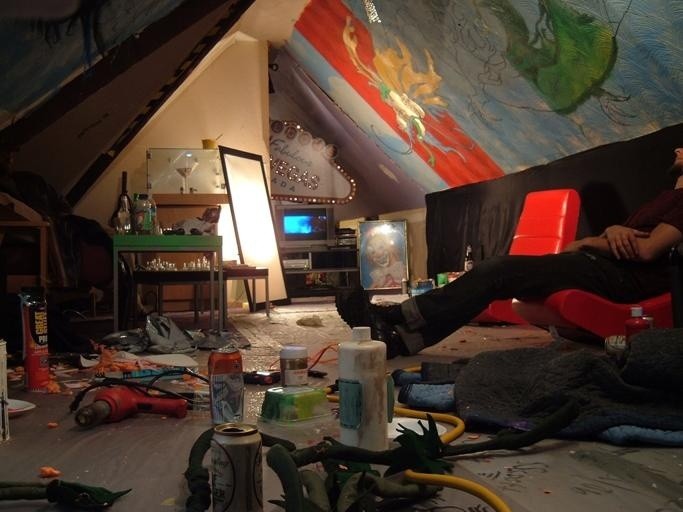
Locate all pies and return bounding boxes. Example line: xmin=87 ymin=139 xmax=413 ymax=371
xmin=216 ymin=134 xmax=223 ymax=141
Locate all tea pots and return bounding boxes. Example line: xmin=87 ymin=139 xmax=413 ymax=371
xmin=218 ymin=145 xmax=291 ymax=313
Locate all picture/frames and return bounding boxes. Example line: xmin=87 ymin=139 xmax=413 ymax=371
xmin=111 ymin=235 xmax=224 ymax=336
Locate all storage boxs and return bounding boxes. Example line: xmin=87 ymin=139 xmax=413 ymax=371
xmin=130 ymin=268 xmax=270 ymax=324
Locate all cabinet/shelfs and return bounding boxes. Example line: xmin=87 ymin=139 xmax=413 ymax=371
xmin=117 ymin=192 xmax=157 ymax=236
xmin=464 ymin=244 xmax=474 ymax=273
xmin=336 ymin=326 xmax=388 ymax=458
xmin=626 ymin=307 xmax=649 ymax=348
xmin=278 ymin=346 xmax=309 ymax=389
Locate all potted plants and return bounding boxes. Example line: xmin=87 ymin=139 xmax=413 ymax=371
xmin=357 ymin=218 xmax=411 ymax=292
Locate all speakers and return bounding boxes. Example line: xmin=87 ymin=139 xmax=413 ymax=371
xmin=335 ymin=284 xmax=408 ymax=360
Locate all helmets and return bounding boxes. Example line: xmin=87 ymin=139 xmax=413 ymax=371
xmin=388 ymin=417 xmax=446 ymax=438
xmin=9 ymin=399 xmax=35 ymax=417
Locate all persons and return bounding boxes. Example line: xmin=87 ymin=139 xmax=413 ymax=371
xmin=335 ymin=145 xmax=683 ymax=361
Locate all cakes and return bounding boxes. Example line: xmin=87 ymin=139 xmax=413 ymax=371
xmin=212 ymin=423 xmax=263 ymax=512
xmin=208 ymin=347 xmax=244 ymax=426
xmin=642 ymin=315 xmax=655 ymax=329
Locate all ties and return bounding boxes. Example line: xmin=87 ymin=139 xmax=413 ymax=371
xmin=274 ymin=204 xmax=337 ymax=250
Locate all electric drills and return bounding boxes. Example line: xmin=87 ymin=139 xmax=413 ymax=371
xmin=75 ymin=380 xmax=188 ymax=426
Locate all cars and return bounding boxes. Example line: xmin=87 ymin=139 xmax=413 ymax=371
xmin=146 ymin=257 xmax=177 ymax=273
xmin=181 ymin=256 xmax=213 ymax=271
